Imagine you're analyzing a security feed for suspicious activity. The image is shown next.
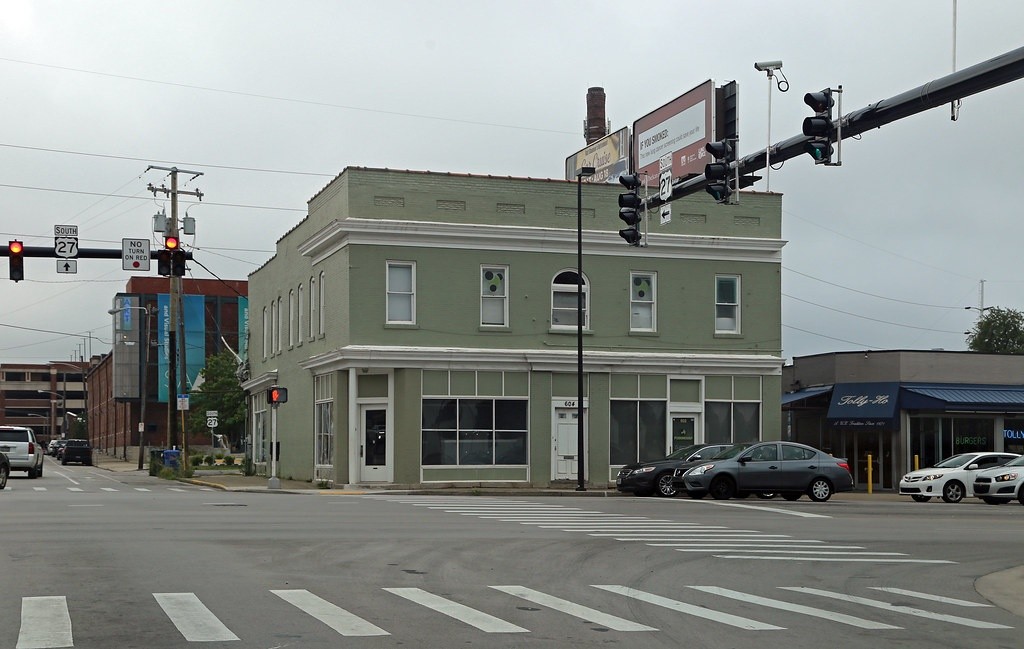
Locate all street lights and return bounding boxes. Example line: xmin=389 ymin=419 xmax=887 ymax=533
xmin=575 ymin=166 xmax=595 ymax=492
xmin=37 ymin=389 xmax=66 ymax=439
xmin=108 ymin=306 xmax=151 ymax=469
xmin=964 ymin=279 xmax=991 ymax=335
xmin=27 ymin=413 xmax=49 ymax=441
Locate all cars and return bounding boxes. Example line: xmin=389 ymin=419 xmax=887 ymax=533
xmin=41 ymin=439 xmax=67 ymax=460
xmin=671 ymin=440 xmax=854 ymax=501
xmin=973 ymin=455 xmax=1024 ymax=505
xmin=898 ymin=452 xmax=1023 ymax=503
xmin=368 ymin=425 xmax=386 ymax=444
xmin=616 ymin=441 xmax=774 ymax=499
xmin=0 ymin=450 xmax=10 ymax=489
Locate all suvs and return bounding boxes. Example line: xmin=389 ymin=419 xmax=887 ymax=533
xmin=61 ymin=439 xmax=92 ymax=466
xmin=0 ymin=425 xmax=44 ymax=480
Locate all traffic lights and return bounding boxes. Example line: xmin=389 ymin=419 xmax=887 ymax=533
xmin=164 ymin=236 xmax=179 ymax=250
xmin=704 ymin=141 xmax=729 ymax=202
xmin=266 ymin=387 xmax=289 ymax=404
xmin=157 ymin=249 xmax=171 ymax=275
xmin=618 ymin=173 xmax=642 ymax=244
xmin=171 ymin=251 xmax=186 ymax=275
xmin=802 ymin=86 xmax=832 ymax=165
xmin=9 ymin=240 xmax=24 ymax=281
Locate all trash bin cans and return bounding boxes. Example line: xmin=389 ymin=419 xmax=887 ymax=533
xmin=149 ymin=450 xmax=165 ymax=476
xmin=164 ymin=449 xmax=180 ymax=473
